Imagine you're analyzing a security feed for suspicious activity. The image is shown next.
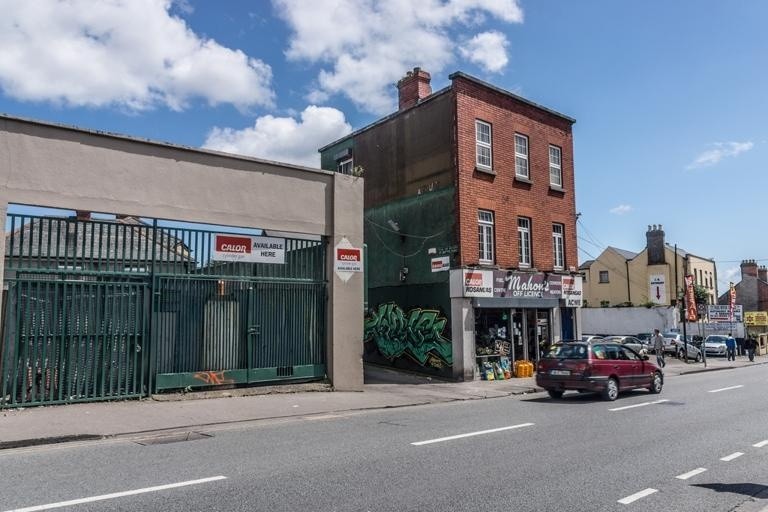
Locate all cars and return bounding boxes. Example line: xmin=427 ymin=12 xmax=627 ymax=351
xmin=682 ymin=334 xmax=738 ymax=357
xmin=582 ymin=335 xmax=652 ymax=354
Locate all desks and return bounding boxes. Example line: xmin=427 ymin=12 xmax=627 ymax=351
xmin=476 ymin=355 xmax=499 ymax=365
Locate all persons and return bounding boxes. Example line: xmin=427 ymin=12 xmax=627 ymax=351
xmin=725 ymin=333 xmax=738 ymax=361
xmin=744 ymin=334 xmax=759 ymax=361
xmin=653 ymin=328 xmax=666 ymax=367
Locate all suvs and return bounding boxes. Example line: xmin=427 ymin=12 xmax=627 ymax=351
xmin=537 ymin=342 xmax=663 ymax=400
xmin=653 ymin=333 xmax=702 ymax=362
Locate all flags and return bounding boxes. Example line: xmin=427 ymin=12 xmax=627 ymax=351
xmin=730 ymin=282 xmax=736 ymax=320
xmin=685 ymin=275 xmax=697 ymax=321
